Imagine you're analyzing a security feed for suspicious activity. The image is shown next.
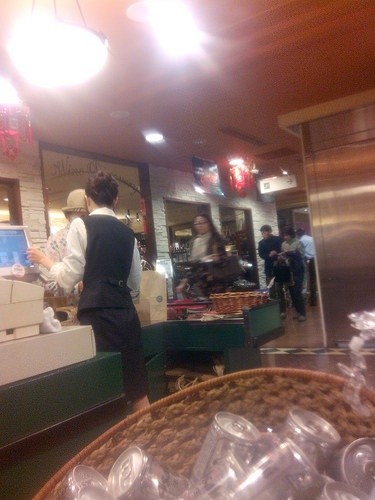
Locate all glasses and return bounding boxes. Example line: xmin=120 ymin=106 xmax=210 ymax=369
xmin=194 ymin=221 xmax=209 ymax=225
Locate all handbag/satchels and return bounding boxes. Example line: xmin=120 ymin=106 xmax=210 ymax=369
xmin=213 ymin=257 xmax=247 ymax=280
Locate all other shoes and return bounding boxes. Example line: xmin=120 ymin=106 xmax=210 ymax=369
xmin=298 ymin=315 xmax=307 ymax=321
xmin=281 ymin=312 xmax=287 ymax=316
xmin=293 ymin=312 xmax=300 ymax=318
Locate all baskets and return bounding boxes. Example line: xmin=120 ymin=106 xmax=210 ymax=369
xmin=32 ymin=367 xmax=375 ymax=500
xmin=210 ymin=290 xmax=268 ymax=313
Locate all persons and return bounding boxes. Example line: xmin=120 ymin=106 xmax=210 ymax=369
xmin=258 ymin=225 xmax=289 ymax=318
xmin=173 ymin=213 xmax=227 ymax=291
xmin=40 ymin=189 xmax=90 ymax=306
xmin=278 ymin=226 xmax=307 ymax=321
xmin=295 ymin=228 xmax=316 ymax=306
xmin=27 ymin=171 xmax=151 ymax=420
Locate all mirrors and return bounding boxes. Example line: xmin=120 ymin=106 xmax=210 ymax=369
xmin=163 ymin=195 xmax=215 ymax=301
xmin=217 ymin=205 xmax=261 ymax=292
xmin=37 ymin=140 xmax=159 ymax=272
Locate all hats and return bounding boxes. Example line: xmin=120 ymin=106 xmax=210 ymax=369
xmin=259 ymin=224 xmax=272 ymax=231
xmin=61 ymin=188 xmax=88 ymax=213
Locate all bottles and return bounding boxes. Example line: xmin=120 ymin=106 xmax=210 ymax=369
xmin=138 ymin=238 xmax=147 ymax=254
xmin=171 ymin=246 xmax=191 ymax=263
